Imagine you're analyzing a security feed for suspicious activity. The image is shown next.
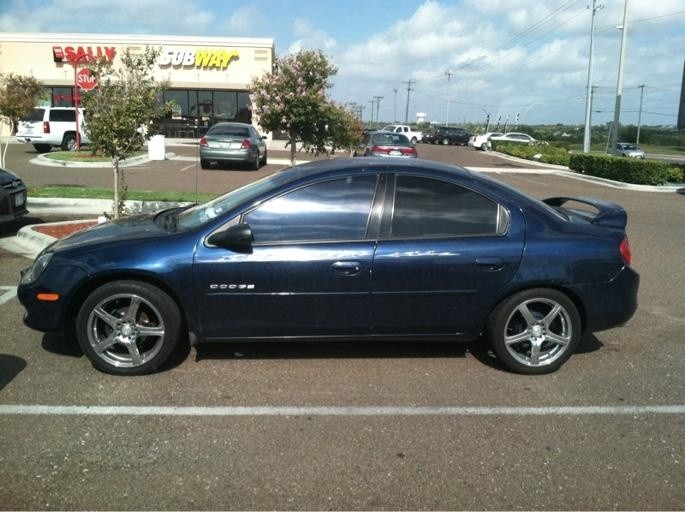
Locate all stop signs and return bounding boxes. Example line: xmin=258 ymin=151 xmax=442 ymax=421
xmin=75 ymin=67 xmax=100 ymax=91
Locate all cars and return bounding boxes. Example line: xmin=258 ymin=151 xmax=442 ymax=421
xmin=198 ymin=120 xmax=270 ymax=172
xmin=16 ymin=149 xmax=641 ymax=380
xmin=1 ymin=167 xmax=30 ymax=224
xmin=606 ymin=142 xmax=647 ymax=160
xmin=354 ymin=130 xmax=418 ymax=160
xmin=375 ymin=122 xmax=550 ymax=152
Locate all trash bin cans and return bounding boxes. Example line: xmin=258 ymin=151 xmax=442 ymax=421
xmin=148 ymin=134 xmax=166 ymax=161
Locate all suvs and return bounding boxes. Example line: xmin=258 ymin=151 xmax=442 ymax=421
xmin=14 ymin=103 xmax=150 ymax=153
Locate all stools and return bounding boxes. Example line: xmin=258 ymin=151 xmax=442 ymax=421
xmin=166 ymin=119 xmax=199 ymax=138
